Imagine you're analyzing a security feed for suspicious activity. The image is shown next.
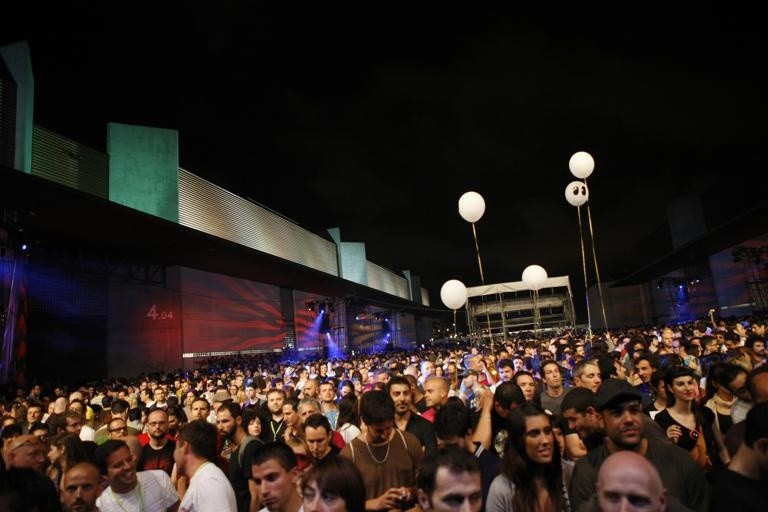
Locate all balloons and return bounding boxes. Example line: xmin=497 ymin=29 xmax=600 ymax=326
xmin=564 ymin=181 xmax=589 ymax=207
xmin=568 ymin=151 xmax=595 ymax=179
xmin=458 ymin=191 xmax=486 ymax=223
xmin=440 ymin=279 xmax=468 ymax=310
xmin=522 ymin=264 xmax=548 ymax=291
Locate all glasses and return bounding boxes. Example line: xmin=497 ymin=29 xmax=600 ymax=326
xmin=13 ymin=435 xmax=48 ymax=450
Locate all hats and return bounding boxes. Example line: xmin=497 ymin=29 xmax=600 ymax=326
xmin=213 ymin=392 xmax=234 ymax=402
xmin=595 ymin=379 xmax=643 ymax=410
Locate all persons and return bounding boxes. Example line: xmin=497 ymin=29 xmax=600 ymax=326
xmin=1 ymin=309 xmax=767 ymax=512
